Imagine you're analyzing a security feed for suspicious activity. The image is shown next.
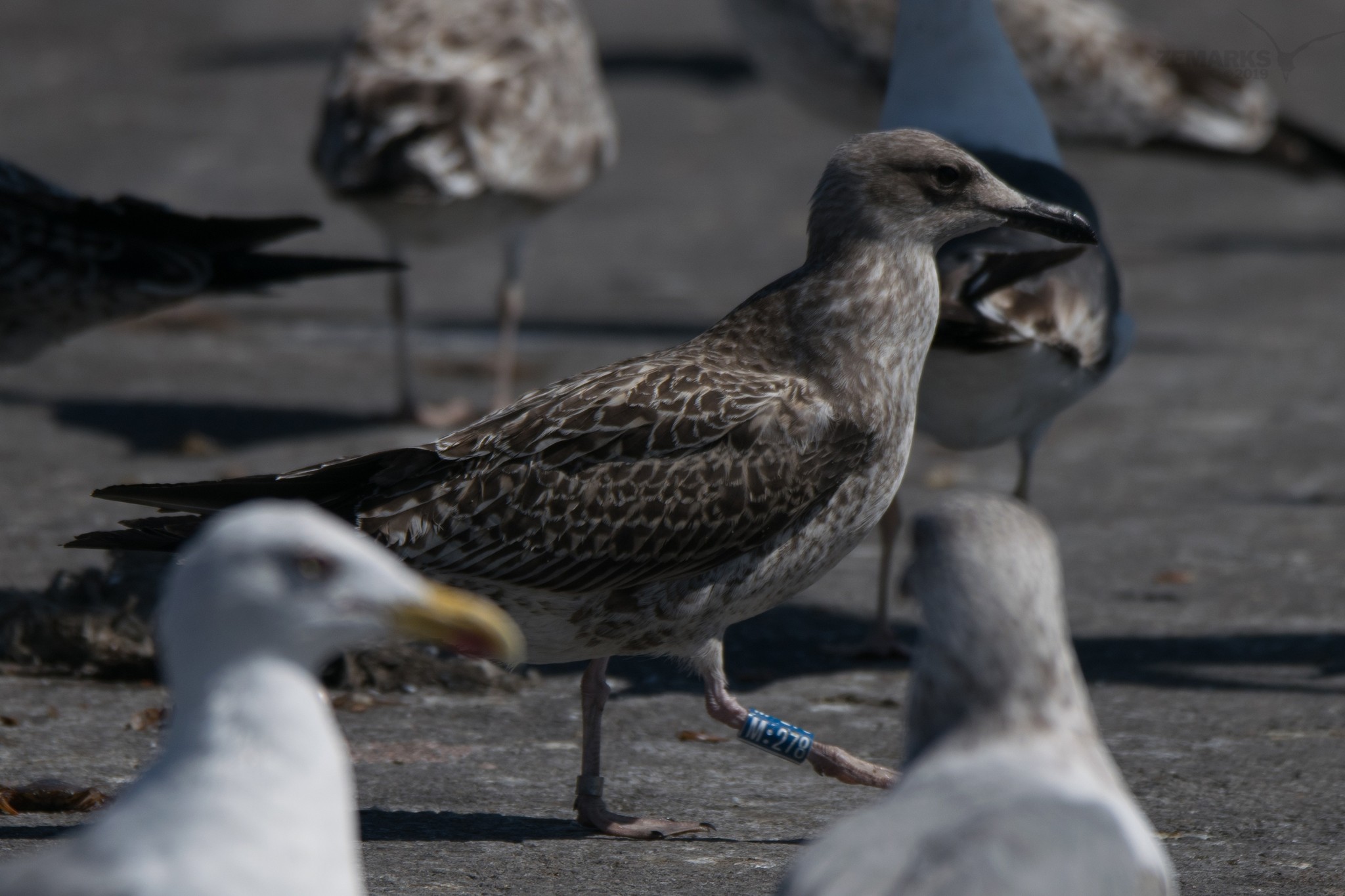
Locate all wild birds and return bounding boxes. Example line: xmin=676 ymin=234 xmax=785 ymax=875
xmin=48 ymin=125 xmax=1099 ymax=847
xmin=0 ymin=156 xmax=408 ymax=378
xmin=789 ymin=0 xmax=1345 ymax=511
xmin=0 ymin=502 xmax=530 ymax=896
xmin=311 ymin=0 xmax=620 ymax=436
xmin=773 ymin=496 xmax=1182 ymax=895
